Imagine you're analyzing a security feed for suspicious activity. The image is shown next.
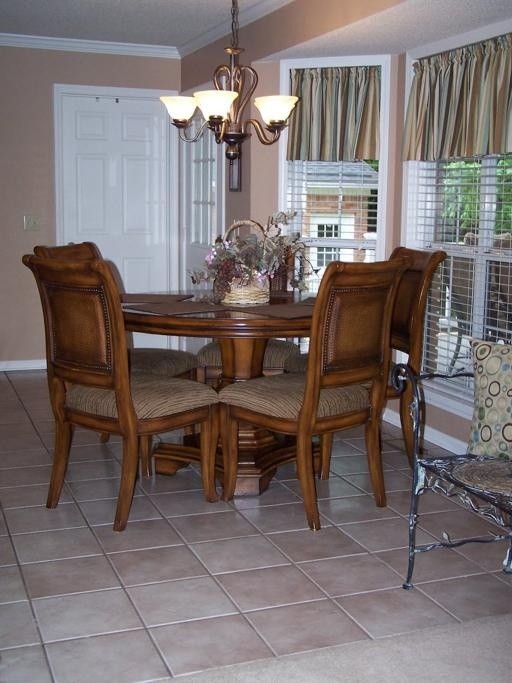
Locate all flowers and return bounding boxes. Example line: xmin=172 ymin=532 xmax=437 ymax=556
xmin=204 ymin=207 xmax=319 ymax=293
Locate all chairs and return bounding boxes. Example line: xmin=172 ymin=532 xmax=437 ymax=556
xmin=21 ymin=252 xmax=218 ymax=532
xmin=218 ymin=251 xmax=414 ymax=532
xmin=193 ymin=234 xmax=301 ymax=384
xmin=31 ymin=237 xmax=201 ymax=442
xmin=280 ymin=243 xmax=447 ymax=470
xmin=388 ymin=360 xmax=511 ymax=592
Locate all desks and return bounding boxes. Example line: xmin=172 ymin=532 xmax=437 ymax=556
xmin=115 ymin=288 xmax=327 ymax=502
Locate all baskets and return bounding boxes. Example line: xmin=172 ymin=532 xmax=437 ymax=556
xmin=217 ymin=218 xmax=272 ymax=308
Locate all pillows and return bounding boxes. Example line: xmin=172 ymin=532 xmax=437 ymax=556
xmin=464 ymin=335 xmax=511 ymax=463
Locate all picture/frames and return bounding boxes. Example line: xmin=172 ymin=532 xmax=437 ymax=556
xmin=228 ymin=142 xmax=244 ymax=192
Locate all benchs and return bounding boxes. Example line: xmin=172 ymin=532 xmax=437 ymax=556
xmin=438 ymin=230 xmax=511 ymax=380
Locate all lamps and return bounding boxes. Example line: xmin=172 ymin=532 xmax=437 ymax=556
xmin=157 ymin=0 xmax=306 ymax=164
